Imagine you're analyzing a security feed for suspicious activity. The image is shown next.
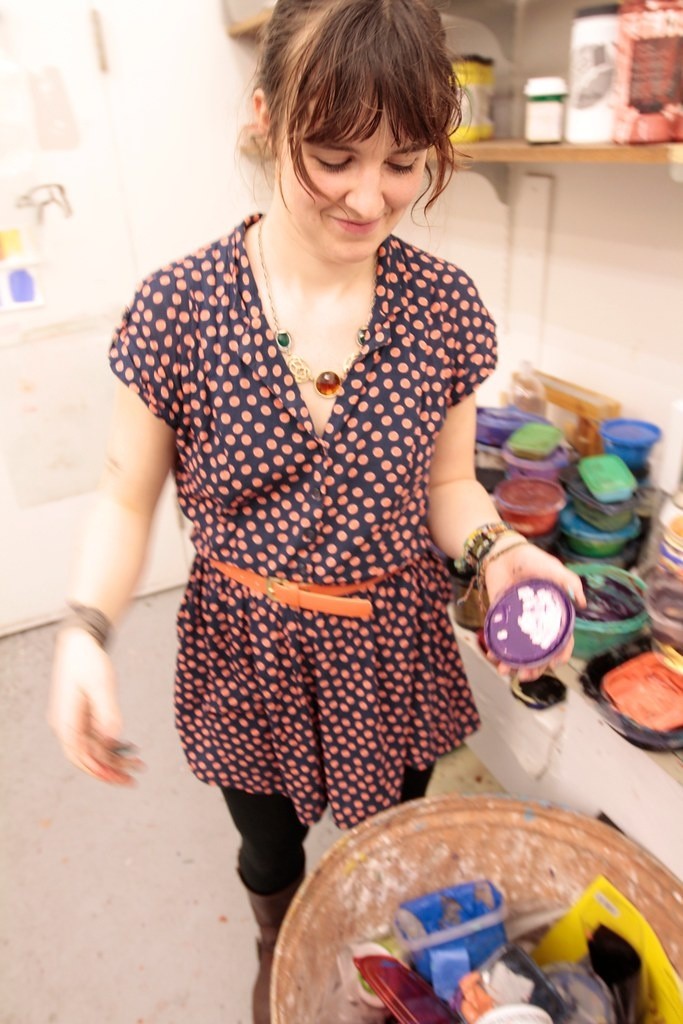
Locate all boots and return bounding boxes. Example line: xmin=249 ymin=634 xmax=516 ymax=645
xmin=237 ymin=846 xmax=306 ymax=1024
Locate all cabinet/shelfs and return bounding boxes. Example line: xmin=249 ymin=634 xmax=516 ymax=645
xmin=226 ymin=0 xmax=683 ymax=208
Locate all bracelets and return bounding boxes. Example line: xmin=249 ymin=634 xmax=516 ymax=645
xmin=76 ymin=607 xmax=110 ymax=644
xmin=455 ymin=520 xmax=528 ymax=613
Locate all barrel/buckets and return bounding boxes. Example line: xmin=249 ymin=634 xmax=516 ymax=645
xmin=444 ymin=555 xmax=488 ymax=630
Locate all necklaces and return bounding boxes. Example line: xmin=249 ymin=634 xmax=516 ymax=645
xmin=259 ymin=223 xmax=377 ymax=397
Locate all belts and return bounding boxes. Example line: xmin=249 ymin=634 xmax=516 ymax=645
xmin=208 ymin=558 xmax=408 ymax=618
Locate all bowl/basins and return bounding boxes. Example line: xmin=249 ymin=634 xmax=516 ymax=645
xmin=564 ymin=562 xmax=649 ymax=660
xmin=472 ymin=442 xmax=505 ymax=494
xmin=563 ymin=470 xmax=639 ymax=532
xmin=600 ymin=418 xmax=661 ymax=469
xmin=475 ymin=407 xmax=551 ymax=446
xmin=495 ymin=479 xmax=567 ymax=536
xmin=559 ymin=506 xmax=642 ymax=557
xmin=501 ymin=446 xmax=567 ymax=484
xmin=579 ymin=636 xmax=683 ymax=753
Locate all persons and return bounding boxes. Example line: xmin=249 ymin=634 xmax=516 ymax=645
xmin=50 ymin=0 xmax=588 ymax=1024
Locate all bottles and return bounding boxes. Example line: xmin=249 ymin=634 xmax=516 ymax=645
xmin=478 ymin=55 xmax=495 ymax=140
xmin=445 ymin=55 xmax=478 ymax=143
xmin=564 ymin=3 xmax=623 ymax=146
